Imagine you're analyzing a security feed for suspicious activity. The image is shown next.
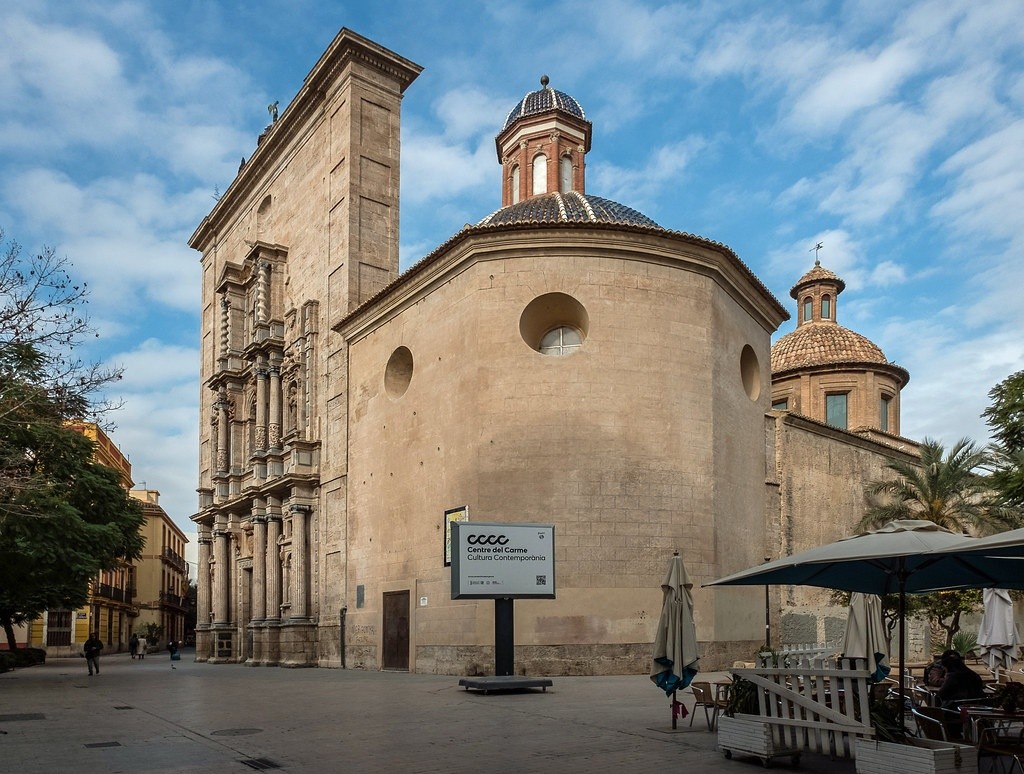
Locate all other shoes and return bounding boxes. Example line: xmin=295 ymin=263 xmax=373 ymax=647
xmin=88 ymin=673 xmax=93 ymax=676
xmin=132 ymin=656 xmax=135 ymax=659
xmin=96 ymin=670 xmax=99 ymax=674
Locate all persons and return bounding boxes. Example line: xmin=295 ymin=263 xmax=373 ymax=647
xmin=169 ymin=641 xmax=182 ymax=669
xmin=83 ymin=633 xmax=104 ymax=676
xmin=930 ymin=650 xmax=982 ymax=703
xmin=129 ymin=633 xmax=147 ymax=659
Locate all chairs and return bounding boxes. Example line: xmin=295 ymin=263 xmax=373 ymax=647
xmin=689 ymin=681 xmax=731 ymax=731
xmin=868 ymin=683 xmax=1024 ymax=774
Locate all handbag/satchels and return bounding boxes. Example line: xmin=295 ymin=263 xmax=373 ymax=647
xmin=144 ymin=645 xmax=148 ymax=650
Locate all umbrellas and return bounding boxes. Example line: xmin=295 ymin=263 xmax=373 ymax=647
xmin=701 ymin=520 xmax=1024 ymax=735
xmin=844 ymin=591 xmax=891 ymax=685
xmin=976 ymin=588 xmax=1021 ymax=681
xmin=649 ymin=549 xmax=700 ymax=730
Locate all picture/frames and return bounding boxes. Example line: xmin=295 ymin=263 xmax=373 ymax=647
xmin=444 ymin=505 xmax=468 ymax=567
